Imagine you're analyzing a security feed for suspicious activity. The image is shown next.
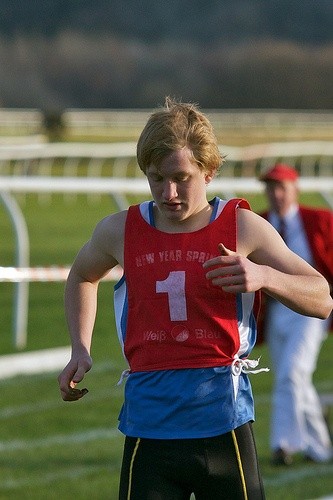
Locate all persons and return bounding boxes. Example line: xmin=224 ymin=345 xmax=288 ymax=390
xmin=243 ymin=163 xmax=333 ymax=468
xmin=56 ymin=98 xmax=333 ymax=499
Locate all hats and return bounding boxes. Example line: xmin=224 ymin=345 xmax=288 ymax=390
xmin=261 ymin=164 xmax=298 ymax=181
